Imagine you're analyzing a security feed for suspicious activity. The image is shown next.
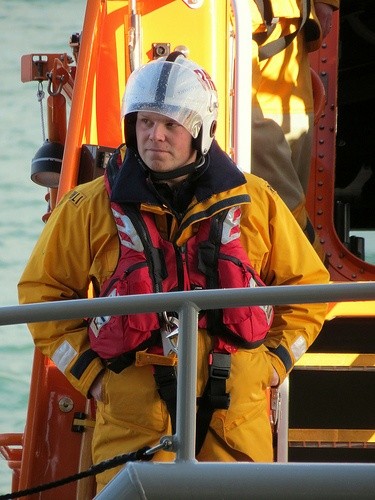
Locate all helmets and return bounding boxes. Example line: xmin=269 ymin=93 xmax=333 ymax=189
xmin=123 ymin=50 xmax=218 ymax=157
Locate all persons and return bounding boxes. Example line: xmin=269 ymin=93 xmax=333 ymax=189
xmin=17 ymin=49 xmax=331 ymax=500
xmin=250 ymin=0 xmax=341 ymax=270
